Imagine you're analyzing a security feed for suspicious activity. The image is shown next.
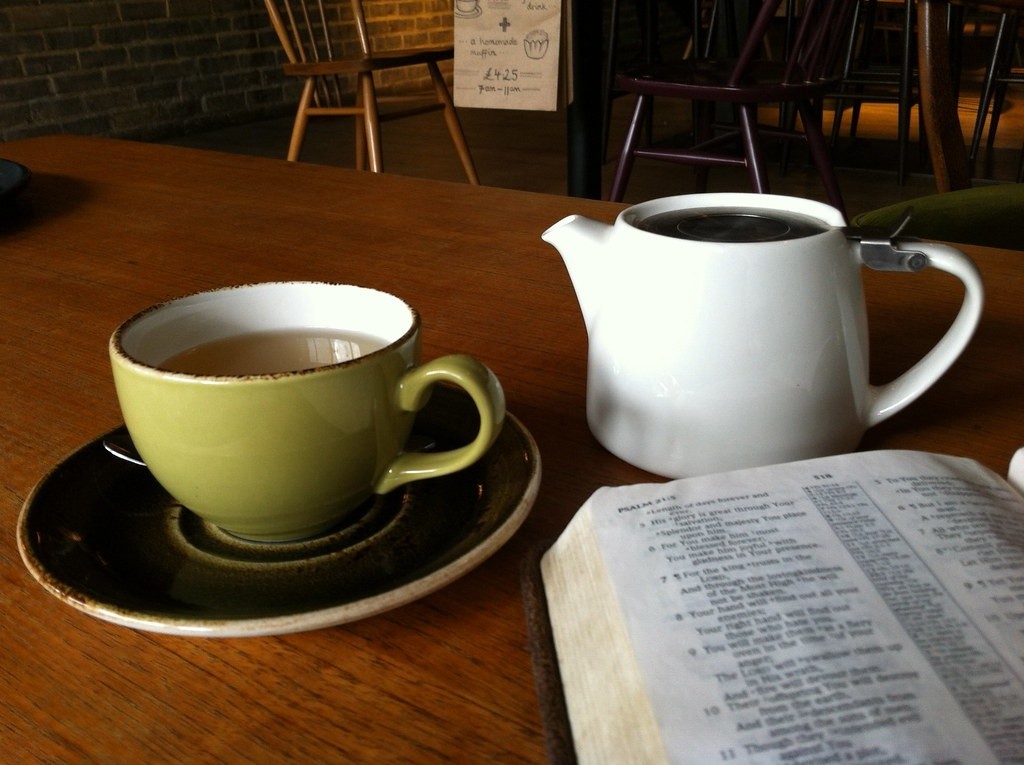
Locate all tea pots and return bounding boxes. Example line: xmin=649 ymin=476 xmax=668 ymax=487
xmin=540 ymin=194 xmax=983 ymax=480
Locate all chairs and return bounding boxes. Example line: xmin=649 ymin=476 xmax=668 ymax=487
xmin=608 ymin=1 xmax=1024 ymax=227
xmin=263 ymin=0 xmax=482 ymax=186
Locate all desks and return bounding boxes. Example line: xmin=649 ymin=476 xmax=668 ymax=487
xmin=0 ymin=136 xmax=1024 ymax=765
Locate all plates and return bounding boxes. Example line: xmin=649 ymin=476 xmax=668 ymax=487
xmin=17 ymin=382 xmax=541 ymax=638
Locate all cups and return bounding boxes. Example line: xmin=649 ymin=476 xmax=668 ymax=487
xmin=109 ymin=282 xmax=505 ymax=542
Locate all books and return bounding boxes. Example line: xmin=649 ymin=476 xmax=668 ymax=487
xmin=540 ymin=447 xmax=1022 ymax=764
